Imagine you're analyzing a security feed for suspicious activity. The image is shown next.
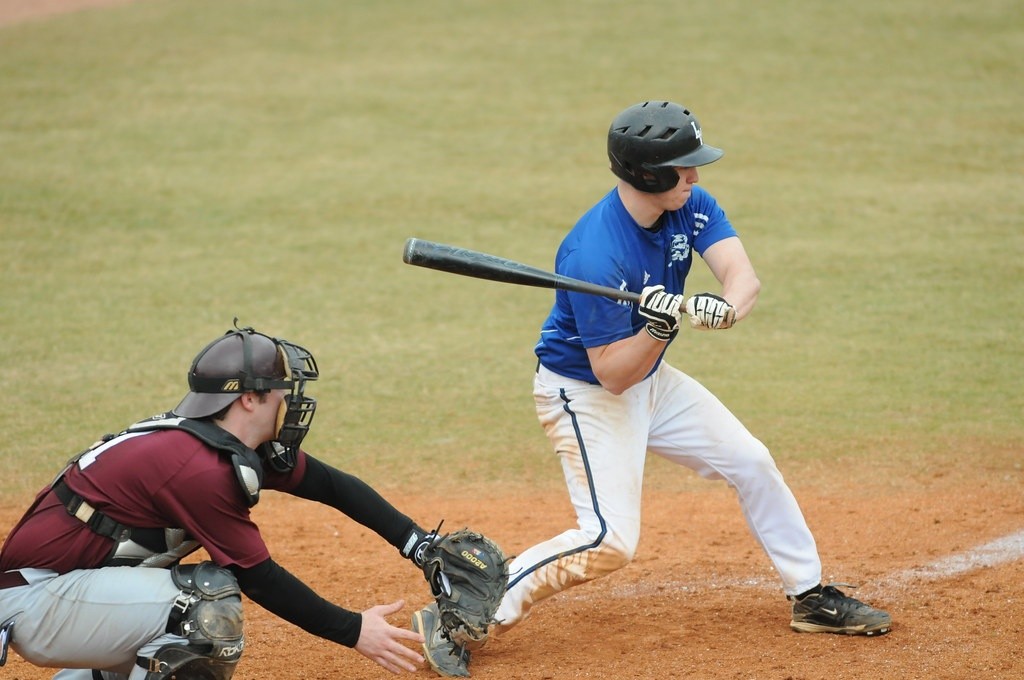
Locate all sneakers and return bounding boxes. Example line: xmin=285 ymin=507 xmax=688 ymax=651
xmin=412 ymin=600 xmax=471 ymax=678
xmin=789 ymin=581 xmax=891 ymax=635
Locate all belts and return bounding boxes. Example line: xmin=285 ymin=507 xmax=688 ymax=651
xmin=0 ymin=570 xmax=28 ymax=589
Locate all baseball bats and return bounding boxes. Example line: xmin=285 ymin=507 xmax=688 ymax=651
xmin=402 ymin=237 xmax=736 ymax=329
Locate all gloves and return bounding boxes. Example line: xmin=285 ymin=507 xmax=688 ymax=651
xmin=685 ymin=291 xmax=737 ymax=329
xmin=637 ymin=284 xmax=683 ymax=342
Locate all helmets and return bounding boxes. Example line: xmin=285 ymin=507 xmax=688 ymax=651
xmin=172 ymin=330 xmax=292 ymax=418
xmin=606 ymin=101 xmax=724 ymax=193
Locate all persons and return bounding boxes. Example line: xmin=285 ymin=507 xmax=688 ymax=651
xmin=0 ymin=315 xmax=511 ymax=679
xmin=411 ymin=100 xmax=893 ymax=680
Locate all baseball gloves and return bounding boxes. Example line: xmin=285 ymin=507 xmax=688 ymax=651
xmin=420 ymin=528 xmax=510 ymax=652
